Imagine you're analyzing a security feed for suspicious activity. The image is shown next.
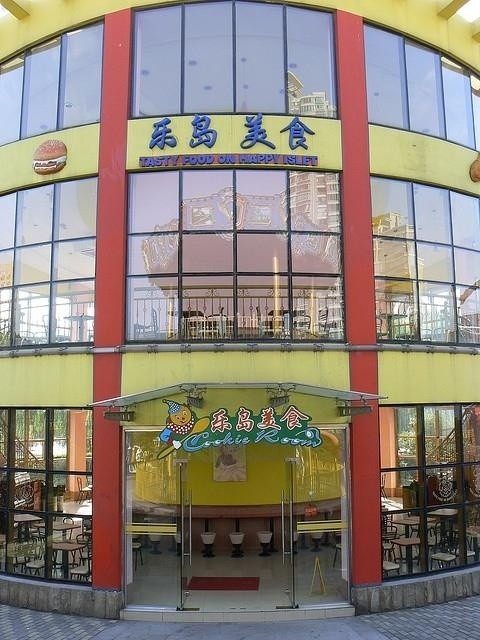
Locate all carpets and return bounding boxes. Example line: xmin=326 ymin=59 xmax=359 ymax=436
xmin=187 ymin=574 xmax=261 ymax=591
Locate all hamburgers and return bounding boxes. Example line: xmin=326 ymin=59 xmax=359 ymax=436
xmin=32 ymin=140 xmax=67 ymax=175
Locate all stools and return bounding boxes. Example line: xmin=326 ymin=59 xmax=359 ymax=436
xmin=145 ymin=528 xmax=326 ymax=565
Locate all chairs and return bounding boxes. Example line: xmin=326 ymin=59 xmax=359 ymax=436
xmin=380 ymin=474 xmax=389 ymax=499
xmin=2 ymin=462 xmax=141 ymax=590
xmin=133 ymin=308 xmax=159 ymax=340
xmin=169 ymin=307 xmax=336 ymax=338
xmin=376 ymin=316 xmax=382 ymax=339
xmin=328 ymin=494 xmax=479 ymax=585
xmin=42 ymin=315 xmax=59 ymax=342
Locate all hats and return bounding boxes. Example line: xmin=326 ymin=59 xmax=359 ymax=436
xmin=161 ymin=398 xmax=180 ymax=414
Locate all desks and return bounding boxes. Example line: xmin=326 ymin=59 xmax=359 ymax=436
xmin=63 ymin=315 xmax=94 ymax=341
xmin=378 ymin=314 xmax=408 ymax=340
